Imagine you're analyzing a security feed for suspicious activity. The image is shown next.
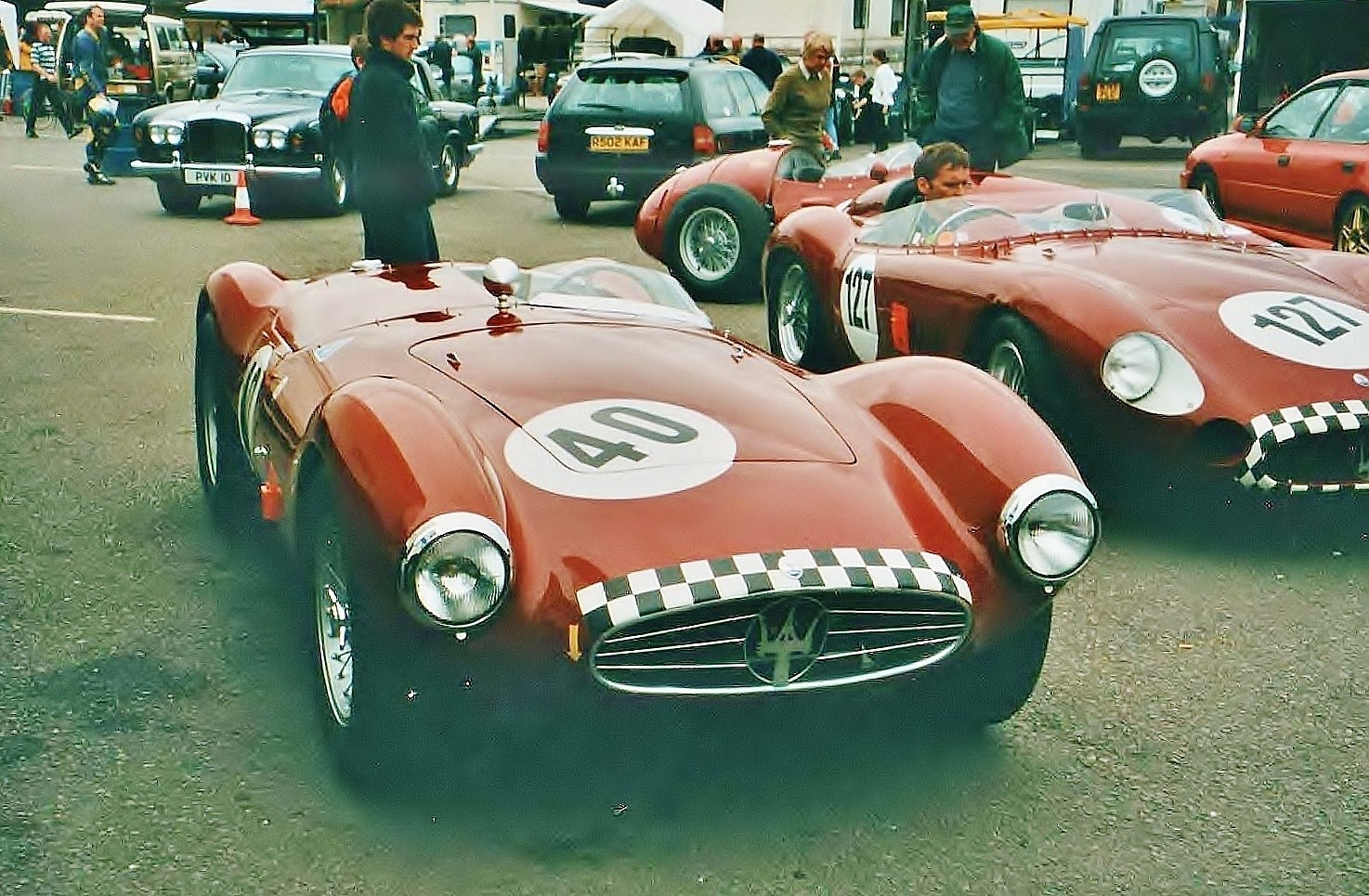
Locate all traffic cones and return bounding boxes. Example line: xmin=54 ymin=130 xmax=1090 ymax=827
xmin=224 ymin=170 xmax=261 ymax=226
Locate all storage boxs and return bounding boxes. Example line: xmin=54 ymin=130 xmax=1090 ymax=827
xmin=126 ymin=65 xmax=151 ymax=79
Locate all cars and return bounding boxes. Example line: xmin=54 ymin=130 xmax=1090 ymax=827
xmin=534 ymin=53 xmax=835 ymax=220
xmin=189 ymin=39 xmax=249 ymax=103
xmin=1179 ymin=71 xmax=1368 ymax=258
xmin=127 ymin=43 xmax=484 ymax=216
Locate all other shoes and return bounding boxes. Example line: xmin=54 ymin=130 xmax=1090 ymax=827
xmin=67 ymin=127 xmax=84 ymax=139
xmin=26 ymin=128 xmax=39 ymax=138
xmin=83 ymin=161 xmax=104 ymax=173
xmin=87 ymin=171 xmax=116 ymax=185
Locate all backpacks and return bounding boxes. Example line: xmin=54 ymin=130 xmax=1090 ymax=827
xmin=318 ymin=71 xmax=357 ymax=146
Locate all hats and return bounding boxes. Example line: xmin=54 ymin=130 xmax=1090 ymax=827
xmin=946 ymin=4 xmax=976 ymax=36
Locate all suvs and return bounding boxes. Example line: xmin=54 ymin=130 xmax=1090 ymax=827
xmin=1075 ymin=13 xmax=1240 ymax=154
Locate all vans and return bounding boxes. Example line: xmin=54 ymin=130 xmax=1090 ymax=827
xmin=18 ymin=1 xmax=206 ymax=123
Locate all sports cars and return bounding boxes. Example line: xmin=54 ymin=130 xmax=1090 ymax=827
xmin=191 ymin=258 xmax=1100 ymax=794
xmin=632 ymin=131 xmax=1278 ymax=310
xmin=757 ymin=172 xmax=1369 ymax=513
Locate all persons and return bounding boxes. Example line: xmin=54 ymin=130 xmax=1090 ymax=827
xmin=910 ymin=143 xmax=974 ymax=242
xmin=345 ymin=0 xmax=440 ymax=270
xmin=1 ymin=1 xmax=900 ymax=189
xmin=759 ymin=30 xmax=833 ymax=166
xmin=908 ymin=3 xmax=1032 ymax=175
